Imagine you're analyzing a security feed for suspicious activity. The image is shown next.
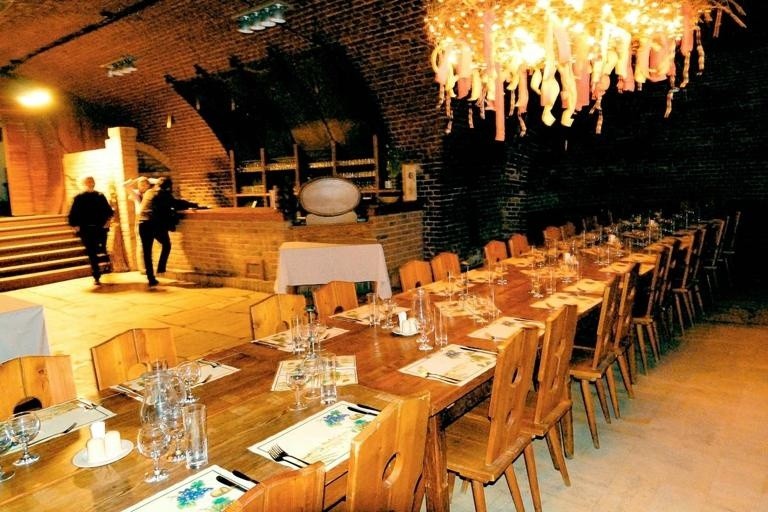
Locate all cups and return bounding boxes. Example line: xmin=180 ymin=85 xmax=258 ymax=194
xmin=530 ymin=270 xmax=545 ymax=298
xmin=7 ymin=411 xmax=41 ymax=467
xmin=545 ymin=239 xmax=558 ymax=266
xmin=162 ymin=407 xmax=186 ymax=463
xmin=303 ymin=359 xmax=320 ymax=400
xmin=381 ymin=298 xmax=397 ymax=329
xmin=90 ymin=421 xmax=106 ymax=439
xmin=401 ymin=320 xmax=410 ymax=333
xmin=290 ymin=315 xmax=307 ymax=353
xmin=496 ymin=262 xmax=509 ymax=285
xmin=137 ymin=421 xmax=170 ymax=483
xmin=182 ymin=404 xmax=207 ymax=470
xmin=474 ymin=284 xmax=490 ymax=325
xmin=449 ymin=265 xmax=463 ymax=311
xmin=0 ymin=420 xmax=15 ymax=482
xmin=414 ymin=312 xmax=435 ymax=352
xmin=487 ymin=256 xmax=498 ymax=284
xmin=304 ymin=321 xmax=320 ymax=361
xmin=409 ymin=318 xmax=418 ymax=333
xmin=319 ymin=351 xmax=337 ymax=405
xmin=286 ymin=363 xmax=308 ymax=411
xmin=88 ymin=437 xmax=106 ymax=461
xmin=542 ymin=266 xmax=556 ymax=294
xmin=105 ymin=430 xmax=121 ymax=451
xmin=367 ymin=292 xmax=381 ymax=326
xmin=559 ymin=209 xmax=701 ymax=291
xmin=434 ymin=306 xmax=448 ymax=347
xmin=176 ymin=360 xmax=201 ymax=406
xmin=412 ymin=288 xmax=431 ymax=343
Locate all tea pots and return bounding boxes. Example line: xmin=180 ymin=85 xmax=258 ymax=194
xmin=139 ymin=370 xmax=187 ymax=429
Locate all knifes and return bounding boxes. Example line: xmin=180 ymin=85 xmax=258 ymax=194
xmin=232 ymin=470 xmax=260 ymax=486
xmin=347 ymin=407 xmax=379 ymax=417
xmin=460 ymin=346 xmax=499 ymax=356
xmin=465 ymin=343 xmax=500 ymax=354
xmin=356 ymin=402 xmax=382 ymax=413
xmin=215 ymin=475 xmax=248 ymax=493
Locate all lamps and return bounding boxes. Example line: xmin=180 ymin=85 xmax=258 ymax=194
xmin=106 ymin=54 xmax=137 ymax=78
xmin=233 ymin=0 xmax=285 ymax=34
xmin=419 ymin=0 xmax=749 ymax=143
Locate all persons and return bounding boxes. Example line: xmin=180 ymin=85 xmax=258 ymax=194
xmin=124 ymin=176 xmax=152 ymax=275
xmin=68 ymin=178 xmax=115 ymax=286
xmin=135 ymin=177 xmax=198 ymax=287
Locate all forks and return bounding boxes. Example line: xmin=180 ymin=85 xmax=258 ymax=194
xmin=417 ymin=369 xmax=457 ymax=386
xmin=197 ymin=374 xmax=212 ymax=384
xmin=420 ymin=366 xmax=462 ymax=382
xmin=270 ymin=443 xmax=311 ymax=466
xmin=197 ymin=359 xmax=218 ymax=369
xmin=27 ymin=421 xmax=77 ymax=447
xmin=268 ymin=443 xmax=310 ymax=468
xmin=202 ymin=357 xmax=222 ymax=366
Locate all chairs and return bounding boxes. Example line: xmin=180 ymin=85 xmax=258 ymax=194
xmin=690 ymin=210 xmax=743 ymax=298
xmin=4 ymin=353 xmax=74 ymax=414
xmin=544 ymin=222 xmax=576 ymax=245
xmin=672 ymin=229 xmax=701 ymax=333
xmin=485 ymin=237 xmax=507 ymax=266
xmin=330 ymin=390 xmax=427 ymax=512
xmin=93 ymin=324 xmax=176 ymax=389
xmin=569 ymin=274 xmax=625 ymax=449
xmin=224 ymin=460 xmax=327 ymax=511
xmin=431 ymin=250 xmax=462 ymax=277
xmin=398 ymin=259 xmax=431 ymax=289
xmin=631 ymin=247 xmax=669 ymax=375
xmin=313 ymin=278 xmax=360 ymax=314
xmin=572 ymin=258 xmax=645 ymax=399
xmin=508 ymin=232 xmax=531 ymax=255
xmin=460 ymin=301 xmax=581 ymax=487
xmin=250 ymin=290 xmax=312 ymax=337
xmin=436 ymin=326 xmax=535 ymax=512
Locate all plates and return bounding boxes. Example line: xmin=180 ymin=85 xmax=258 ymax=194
xmin=69 ymin=439 xmax=135 ymax=468
xmin=392 ymin=327 xmax=420 ymax=337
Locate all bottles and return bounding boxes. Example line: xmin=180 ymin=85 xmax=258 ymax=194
xmin=385 ymin=162 xmax=393 ymax=188
